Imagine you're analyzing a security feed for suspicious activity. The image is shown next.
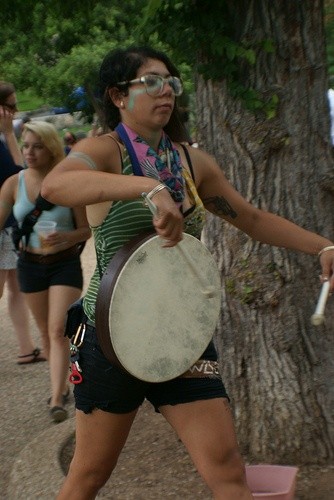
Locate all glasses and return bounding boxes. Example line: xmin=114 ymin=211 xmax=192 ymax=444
xmin=0 ymin=101 xmax=17 ymax=111
xmin=117 ymin=74 xmax=184 ymax=97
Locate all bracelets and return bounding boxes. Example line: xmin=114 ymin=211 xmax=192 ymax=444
xmin=143 ymin=183 xmax=171 ymax=207
xmin=318 ymin=245 xmax=334 ymax=256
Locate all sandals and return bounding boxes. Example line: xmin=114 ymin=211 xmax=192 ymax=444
xmin=17 ymin=347 xmax=48 ymax=365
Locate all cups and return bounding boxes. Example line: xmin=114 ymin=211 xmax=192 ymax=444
xmin=35 ymin=221 xmax=57 ymax=251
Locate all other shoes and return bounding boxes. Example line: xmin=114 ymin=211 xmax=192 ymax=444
xmin=47 ymin=385 xmax=70 ymax=406
xmin=49 ymin=406 xmax=68 ymax=423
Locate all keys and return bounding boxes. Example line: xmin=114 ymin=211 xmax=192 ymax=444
xmin=70 ymin=350 xmax=83 ymax=373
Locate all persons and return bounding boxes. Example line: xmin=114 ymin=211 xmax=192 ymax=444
xmin=41 ymin=43 xmax=334 ymax=500
xmin=0 ymin=80 xmax=92 ymax=424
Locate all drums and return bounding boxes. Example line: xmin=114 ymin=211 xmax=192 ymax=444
xmin=92 ymin=230 xmax=223 ymax=383
